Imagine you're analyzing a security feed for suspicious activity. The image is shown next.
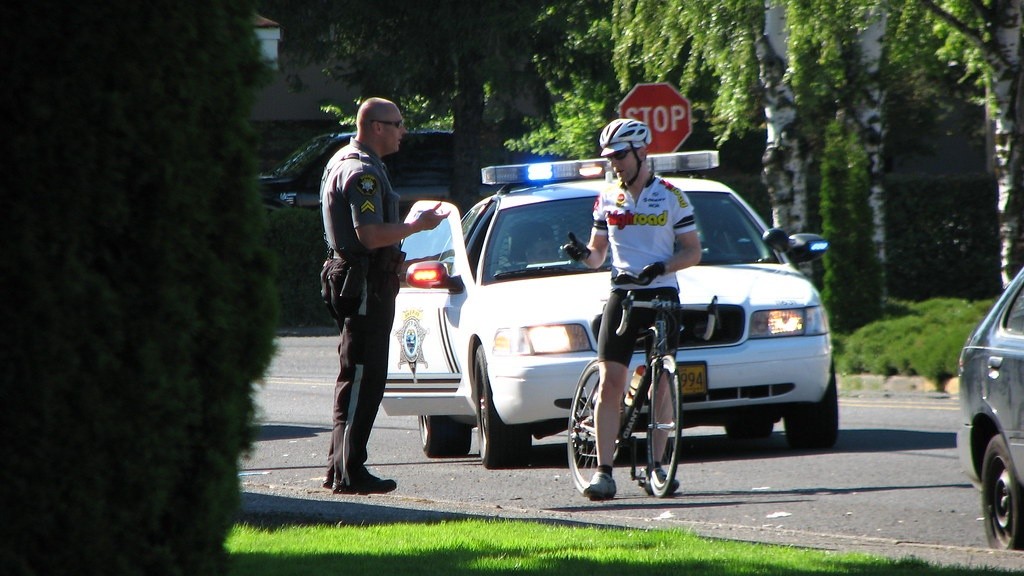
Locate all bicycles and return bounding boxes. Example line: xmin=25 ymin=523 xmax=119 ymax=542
xmin=564 ymin=293 xmax=721 ymax=499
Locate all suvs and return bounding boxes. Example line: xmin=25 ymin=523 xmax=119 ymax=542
xmin=253 ymin=127 xmax=510 ymax=224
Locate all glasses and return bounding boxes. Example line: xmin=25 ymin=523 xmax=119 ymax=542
xmin=604 ymin=148 xmax=641 ymax=162
xmin=530 ymin=234 xmax=551 ymax=243
xmin=370 ymin=118 xmax=406 ymax=130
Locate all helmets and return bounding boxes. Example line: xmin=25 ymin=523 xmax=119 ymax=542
xmin=598 ymin=119 xmax=652 ymax=148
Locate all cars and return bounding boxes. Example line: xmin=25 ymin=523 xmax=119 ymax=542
xmin=954 ymin=267 xmax=1024 ymax=562
xmin=377 ymin=146 xmax=843 ymax=469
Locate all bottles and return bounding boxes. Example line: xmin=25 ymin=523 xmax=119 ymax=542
xmin=624 ymin=365 xmax=646 ymax=405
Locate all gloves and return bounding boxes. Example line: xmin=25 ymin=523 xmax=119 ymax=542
xmin=638 ymin=263 xmax=664 ymax=286
xmin=563 ymin=232 xmax=592 ymax=262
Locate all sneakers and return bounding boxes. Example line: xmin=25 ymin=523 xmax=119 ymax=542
xmin=642 ymin=470 xmax=680 ymax=497
xmin=323 ymin=467 xmax=399 ymax=496
xmin=583 ymin=471 xmax=617 ymax=501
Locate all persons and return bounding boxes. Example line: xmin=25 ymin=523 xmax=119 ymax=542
xmin=563 ymin=118 xmax=702 ymax=502
xmin=319 ymin=98 xmax=452 ymax=495
xmin=525 ymin=232 xmax=560 ymax=263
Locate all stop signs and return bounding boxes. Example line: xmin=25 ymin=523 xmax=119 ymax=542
xmin=615 ymin=81 xmax=692 ymax=158
